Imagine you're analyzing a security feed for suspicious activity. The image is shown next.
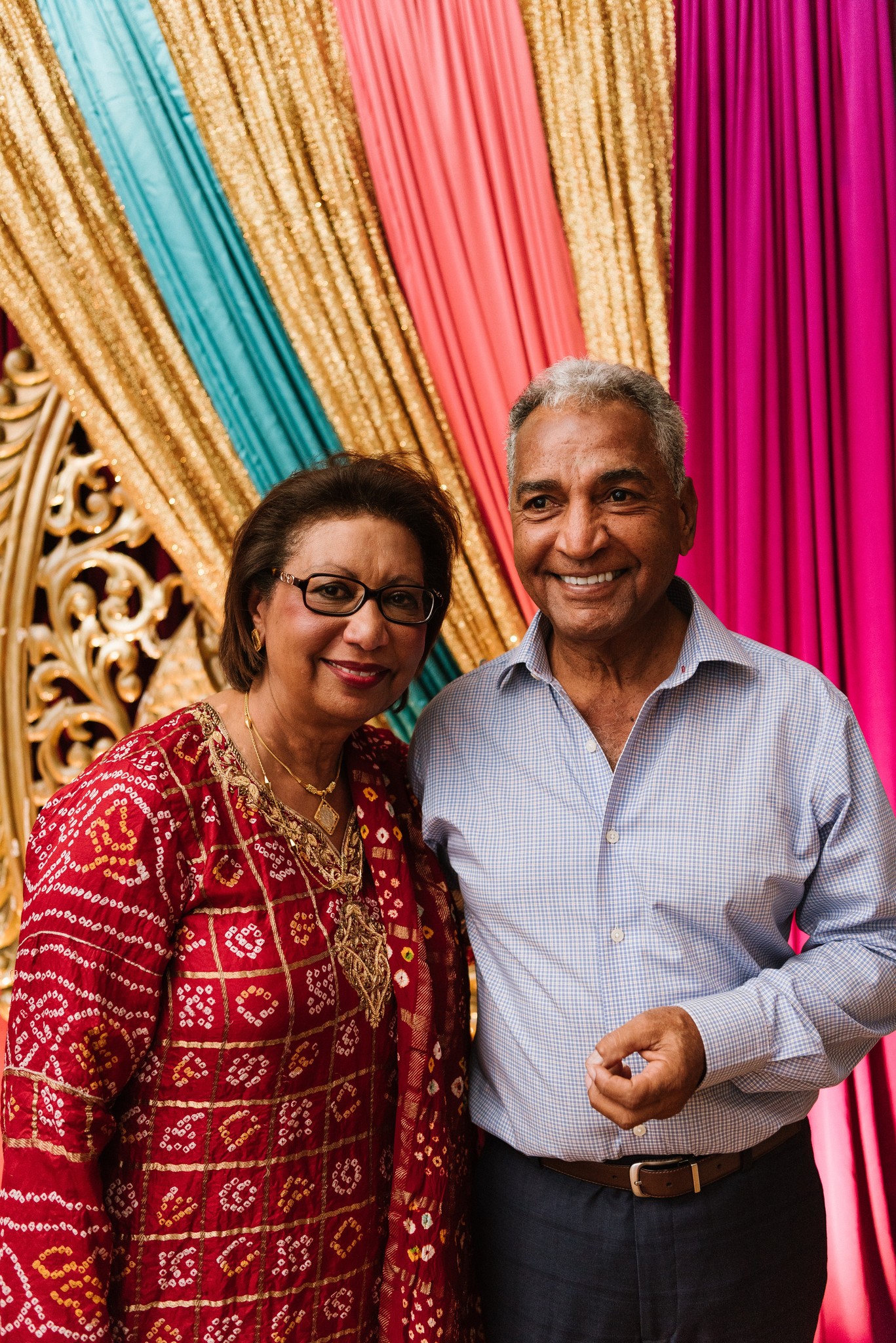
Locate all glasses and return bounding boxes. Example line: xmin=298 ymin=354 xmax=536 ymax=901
xmin=266 ymin=563 xmax=444 ymax=629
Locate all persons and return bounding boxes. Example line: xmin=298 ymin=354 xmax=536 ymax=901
xmin=402 ymin=356 xmax=894 ymax=1342
xmin=1 ymin=450 xmax=487 ymax=1343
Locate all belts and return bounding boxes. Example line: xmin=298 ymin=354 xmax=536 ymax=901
xmin=479 ymin=1114 xmax=809 ymax=1201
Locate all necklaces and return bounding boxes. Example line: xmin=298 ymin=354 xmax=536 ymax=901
xmin=242 ymin=689 xmax=395 ymax=1030
